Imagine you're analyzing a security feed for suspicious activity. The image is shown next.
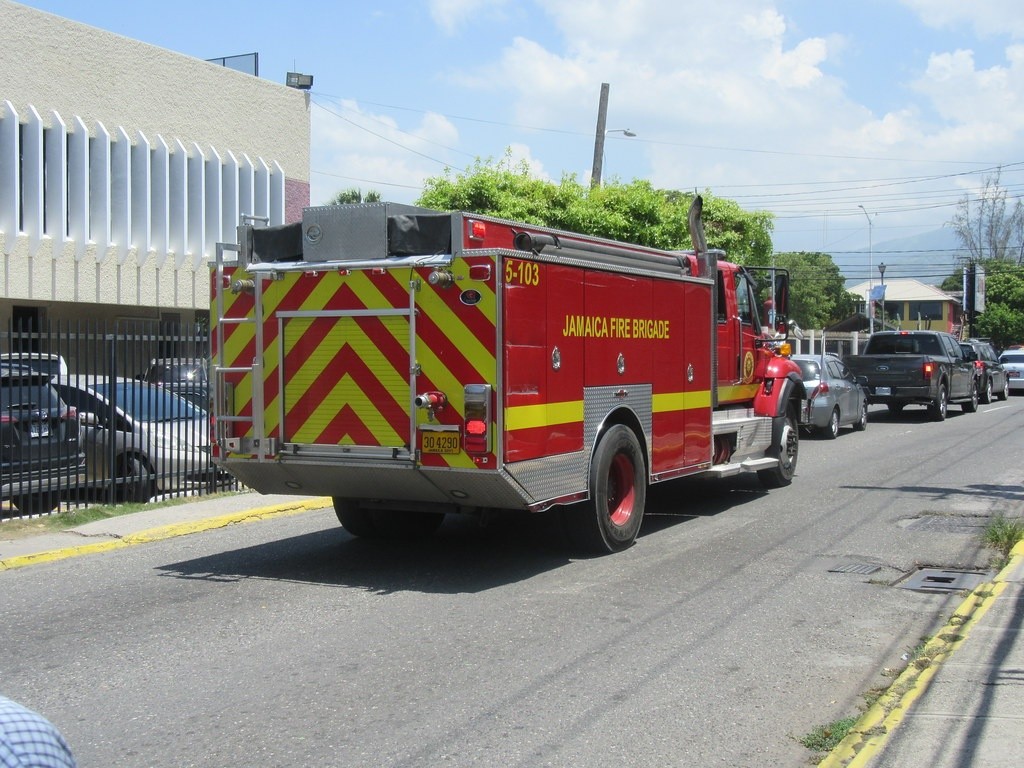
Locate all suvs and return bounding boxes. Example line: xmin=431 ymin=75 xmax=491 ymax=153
xmin=959 ymin=338 xmax=1010 ymax=404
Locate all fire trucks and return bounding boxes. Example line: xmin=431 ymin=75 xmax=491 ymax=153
xmin=206 ymin=192 xmax=806 ymax=558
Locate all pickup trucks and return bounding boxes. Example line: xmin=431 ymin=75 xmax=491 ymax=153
xmin=840 ymin=331 xmax=978 ymax=421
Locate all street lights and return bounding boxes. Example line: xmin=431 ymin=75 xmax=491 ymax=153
xmin=858 ymin=201 xmax=878 ymax=292
xmin=876 ymin=263 xmax=887 ymax=333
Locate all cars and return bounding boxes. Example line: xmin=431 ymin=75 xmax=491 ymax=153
xmin=785 ymin=353 xmax=868 ymax=439
xmin=998 ymin=349 xmax=1024 ymax=392
xmin=2 ymin=321 xmax=219 ymax=522
